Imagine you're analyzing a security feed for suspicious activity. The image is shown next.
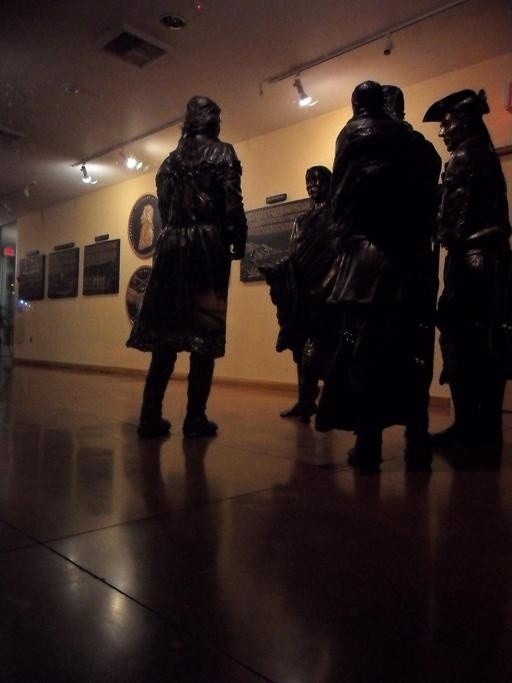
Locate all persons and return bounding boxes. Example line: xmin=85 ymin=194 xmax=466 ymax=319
xmin=256 ymin=82 xmax=512 ymax=451
xmin=126 ymin=82 xmax=247 ymax=435
